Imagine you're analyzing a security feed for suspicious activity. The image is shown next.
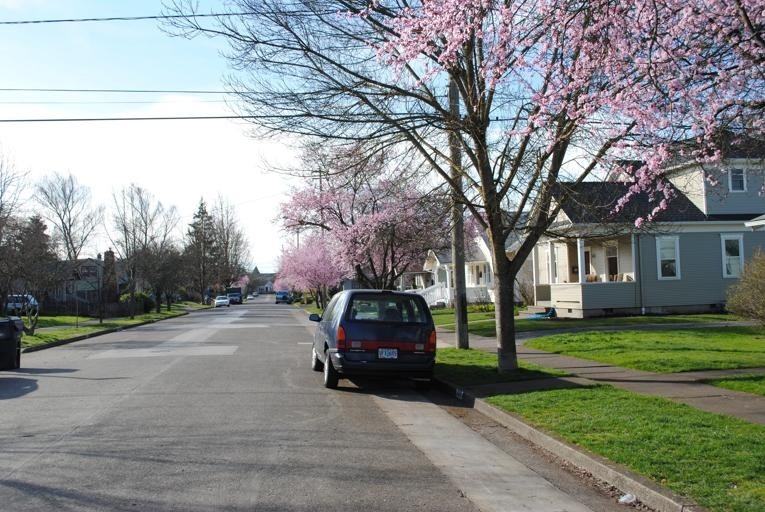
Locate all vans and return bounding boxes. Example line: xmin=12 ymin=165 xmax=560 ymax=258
xmin=306 ymin=289 xmax=438 ymax=392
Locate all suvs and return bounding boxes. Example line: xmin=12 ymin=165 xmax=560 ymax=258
xmin=2 ymin=294 xmax=38 ymax=317
xmin=274 ymin=290 xmax=290 ymax=304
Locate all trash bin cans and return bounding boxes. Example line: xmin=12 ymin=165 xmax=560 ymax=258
xmin=0 ymin=316 xmax=23 ymax=371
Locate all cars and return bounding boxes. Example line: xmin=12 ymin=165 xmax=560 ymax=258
xmin=215 ymin=286 xmax=259 ymax=309
